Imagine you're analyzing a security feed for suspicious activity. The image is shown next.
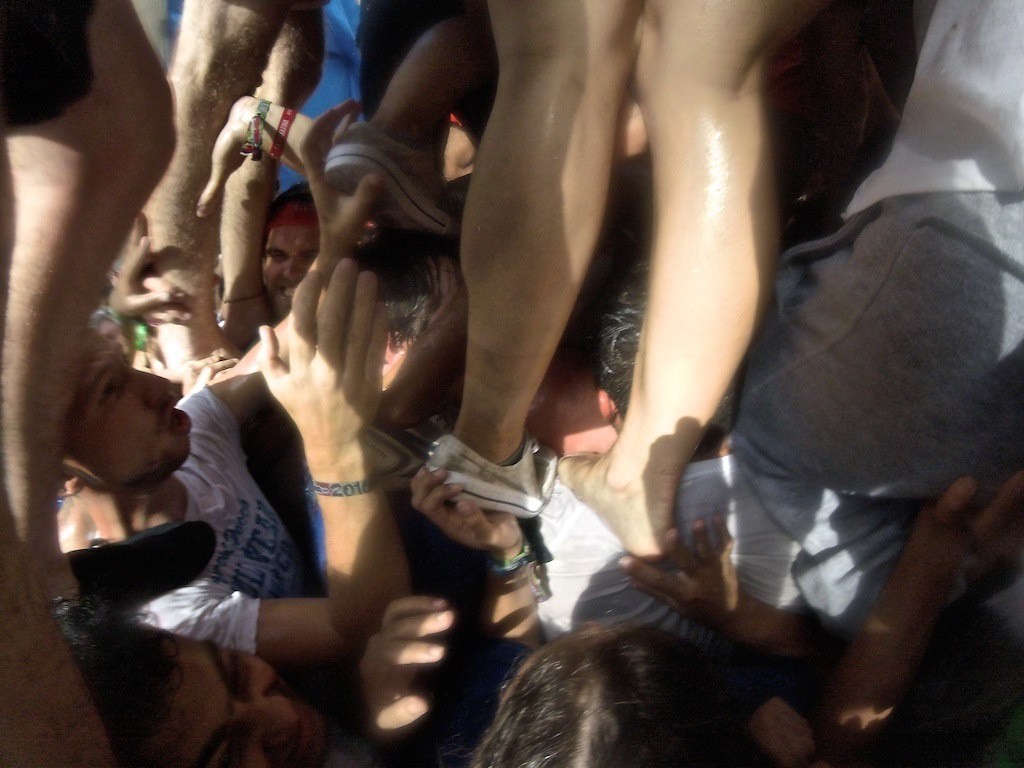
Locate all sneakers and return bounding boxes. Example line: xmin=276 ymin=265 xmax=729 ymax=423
xmin=426 ymin=426 xmax=558 ymax=519
xmin=323 ymin=120 xmax=461 ymax=236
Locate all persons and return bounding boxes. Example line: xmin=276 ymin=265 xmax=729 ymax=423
xmin=0 ymin=0 xmax=1020 ymax=767
